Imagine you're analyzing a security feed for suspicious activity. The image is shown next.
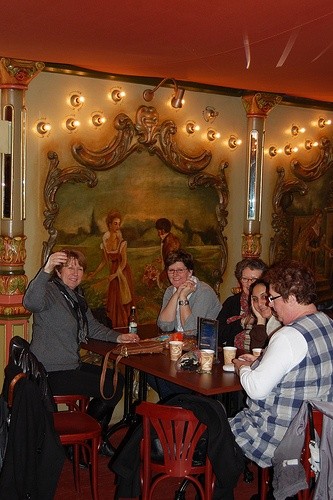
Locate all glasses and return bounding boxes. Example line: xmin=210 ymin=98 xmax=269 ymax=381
xmin=62 ymin=264 xmax=84 ymax=274
xmin=267 ymin=295 xmax=282 ymax=303
xmin=240 ymin=277 xmax=259 ymax=283
xmin=167 ymin=268 xmax=188 ymax=274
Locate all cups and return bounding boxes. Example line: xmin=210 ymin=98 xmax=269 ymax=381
xmin=252 ymin=348 xmax=264 ymax=356
xmin=169 ymin=340 xmax=183 ymax=361
xmin=200 ymin=348 xmax=215 ymax=371
xmin=223 ymin=347 xmax=237 ymax=365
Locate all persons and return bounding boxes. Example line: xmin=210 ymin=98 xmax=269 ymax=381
xmin=146 ymin=250 xmax=333 ymax=500
xmin=22 ymin=249 xmax=139 ymax=468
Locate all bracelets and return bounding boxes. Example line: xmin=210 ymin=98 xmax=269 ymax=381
xmin=178 ymin=300 xmax=189 ymax=305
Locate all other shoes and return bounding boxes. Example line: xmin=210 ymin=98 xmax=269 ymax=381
xmin=65 ymin=438 xmax=116 ymax=469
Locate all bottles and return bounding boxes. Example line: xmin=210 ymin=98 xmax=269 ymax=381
xmin=128 ymin=306 xmax=137 ymax=333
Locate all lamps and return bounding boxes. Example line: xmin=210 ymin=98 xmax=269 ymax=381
xmin=142 ymin=77 xmax=186 ymax=108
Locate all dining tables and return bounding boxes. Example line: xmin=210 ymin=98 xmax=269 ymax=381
xmin=79 ymin=325 xmax=265 ymax=500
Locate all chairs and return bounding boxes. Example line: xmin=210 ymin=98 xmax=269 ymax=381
xmin=48 ymin=372 xmax=102 ymax=500
xmin=261 ymin=410 xmax=323 ymax=500
xmin=135 ymin=402 xmax=216 ymax=500
